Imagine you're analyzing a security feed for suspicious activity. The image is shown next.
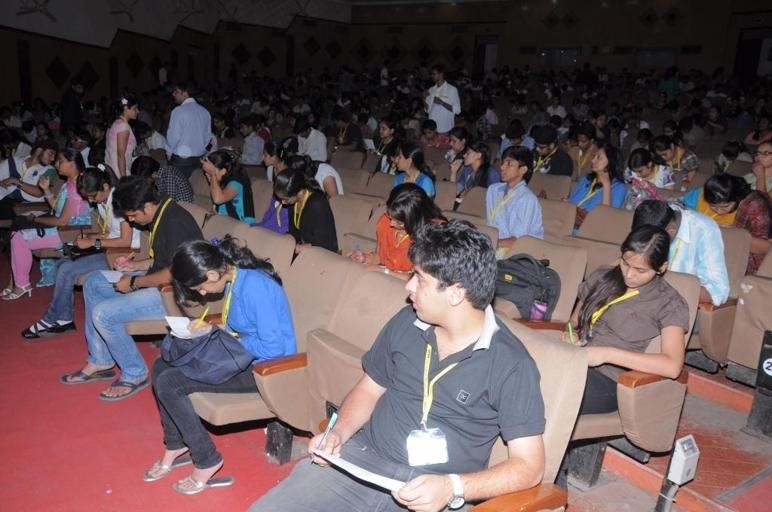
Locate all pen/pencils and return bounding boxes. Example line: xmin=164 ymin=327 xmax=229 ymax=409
xmin=194 ymin=306 xmax=210 ymax=330
xmin=311 ymin=412 xmax=338 ymax=463
xmin=80 ymin=227 xmax=84 ymax=239
xmin=119 ymin=250 xmax=135 ymax=267
xmin=569 ymin=323 xmax=575 ymax=346
xmin=354 ymin=243 xmax=360 ymax=253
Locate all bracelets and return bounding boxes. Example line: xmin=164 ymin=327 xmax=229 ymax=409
xmin=385 ymin=268 xmax=389 ymax=274
xmin=45 ymin=193 xmax=54 ymax=199
xmin=31 ymin=217 xmax=35 ymax=223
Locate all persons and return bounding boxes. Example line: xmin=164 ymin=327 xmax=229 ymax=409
xmin=308 ymin=114 xmax=324 ymax=132
xmin=422 ymin=123 xmax=442 ymax=154
xmin=0 ymin=149 xmax=91 ymax=301
xmin=0 ymin=140 xmax=59 ymax=219
xmin=131 ymin=155 xmax=195 ymax=205
xmin=560 ymin=144 xmax=627 ymax=239
xmin=285 ymin=117 xmax=328 ymax=163
xmin=200 ymin=151 xmax=253 ymax=224
xmin=210 ymin=112 xmax=239 ymax=151
xmin=651 ymin=135 xmax=700 ymax=182
xmin=363 ymin=118 xmax=401 ymax=175
xmin=567 ymin=123 xmax=599 ymax=182
xmin=22 ymin=163 xmax=141 ymax=339
xmin=273 ymin=167 xmax=334 ymax=265
xmin=234 ymin=117 xmax=265 ymax=166
xmin=255 ymin=114 xmax=270 ymax=143
xmin=444 ymin=128 xmax=468 ymax=184
xmin=530 ymin=126 xmax=570 ymax=181
xmin=717 ymin=141 xmax=754 ymax=175
xmin=106 ymin=94 xmax=140 ymax=180
xmin=249 ymin=155 xmax=308 ymax=233
xmin=60 ymin=194 xmax=204 ymax=402
xmin=381 ymin=140 xmax=405 ymax=176
xmin=456 ymin=65 xmax=631 ymax=139
xmin=135 ymin=86 xmax=172 ymax=125
xmin=486 ymin=147 xmax=545 ymax=259
xmin=143 ymin=236 xmax=297 ymax=495
xmin=391 ymin=144 xmax=435 ymax=200
xmin=247 ymin=220 xmax=546 ymax=512
xmin=452 ymin=142 xmax=495 ymax=211
xmin=167 ymin=79 xmax=217 ymax=180
xmin=262 ymin=140 xmax=287 ymax=183
xmin=0 ymin=78 xmax=110 ymax=167
xmin=634 ymin=199 xmax=728 ymax=332
xmin=288 ymin=113 xmax=299 ymax=131
xmin=306 ymin=161 xmax=346 ymax=199
xmin=196 ymin=68 xmax=351 ymax=116
xmin=425 ymin=66 xmax=457 ymax=135
xmin=741 ymin=140 xmax=772 ymax=197
xmin=133 ymin=121 xmax=172 ymax=163
xmin=328 ymin=107 xmax=359 ymax=154
xmin=623 ymin=149 xmax=677 ymax=189
xmin=346 ymin=182 xmax=447 ymax=281
xmin=632 ymin=67 xmax=772 ymax=145
xmin=351 ymin=60 xmax=432 ymax=126
xmin=556 ymin=225 xmax=687 ymax=493
xmin=681 ymin=174 xmax=772 ymax=278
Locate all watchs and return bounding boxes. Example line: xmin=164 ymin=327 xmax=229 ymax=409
xmin=17 ymin=182 xmax=24 ymax=188
xmin=96 ymin=238 xmax=101 ymax=249
xmin=447 ymin=473 xmax=465 ymax=510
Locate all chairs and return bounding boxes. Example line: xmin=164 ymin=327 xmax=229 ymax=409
xmin=685 ymin=227 xmax=754 ymax=372
xmin=319 ymin=311 xmax=589 ymax=511
xmin=33 ymin=222 xmax=99 ymax=259
xmin=570 ymin=202 xmax=636 ymax=280
xmin=201 ymin=215 xmax=250 ymax=240
xmin=188 ymin=248 xmax=366 ymax=465
xmin=535 ymin=197 xmax=578 ymax=242
xmin=126 ymin=227 xmax=295 ymax=352
xmin=306 ymin=268 xmax=412 ymax=406
xmin=456 ymin=186 xmax=488 ymax=216
xmin=189 ymin=174 xmax=216 ymax=211
xmin=570 ymin=257 xmax=701 ymax=492
xmin=254 ymin=147 xmax=455 ymax=266
xmin=76 ymin=200 xmax=211 ymax=287
xmin=526 ymin=173 xmax=570 ymax=200
xmin=493 ymin=235 xmax=586 ymax=343
xmin=727 ymin=244 xmax=772 ymax=385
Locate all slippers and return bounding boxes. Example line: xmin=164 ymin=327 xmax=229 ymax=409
xmin=175 ymin=475 xmax=234 ymax=494
xmin=60 ymin=367 xmax=117 ymax=384
xmin=143 ymin=454 xmax=194 ymax=482
xmin=100 ymin=377 xmax=150 ymax=403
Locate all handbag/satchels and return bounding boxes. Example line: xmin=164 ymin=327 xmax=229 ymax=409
xmin=158 ymin=326 xmax=257 ymax=384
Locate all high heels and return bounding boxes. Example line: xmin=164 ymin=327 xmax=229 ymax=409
xmin=2 ymin=284 xmax=33 ymax=300
xmin=0 ymin=287 xmax=13 ymax=296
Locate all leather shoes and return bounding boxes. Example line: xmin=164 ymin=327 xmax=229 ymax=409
xmin=38 ymin=322 xmax=80 ymax=338
xmin=20 ymin=318 xmax=60 ymax=339
